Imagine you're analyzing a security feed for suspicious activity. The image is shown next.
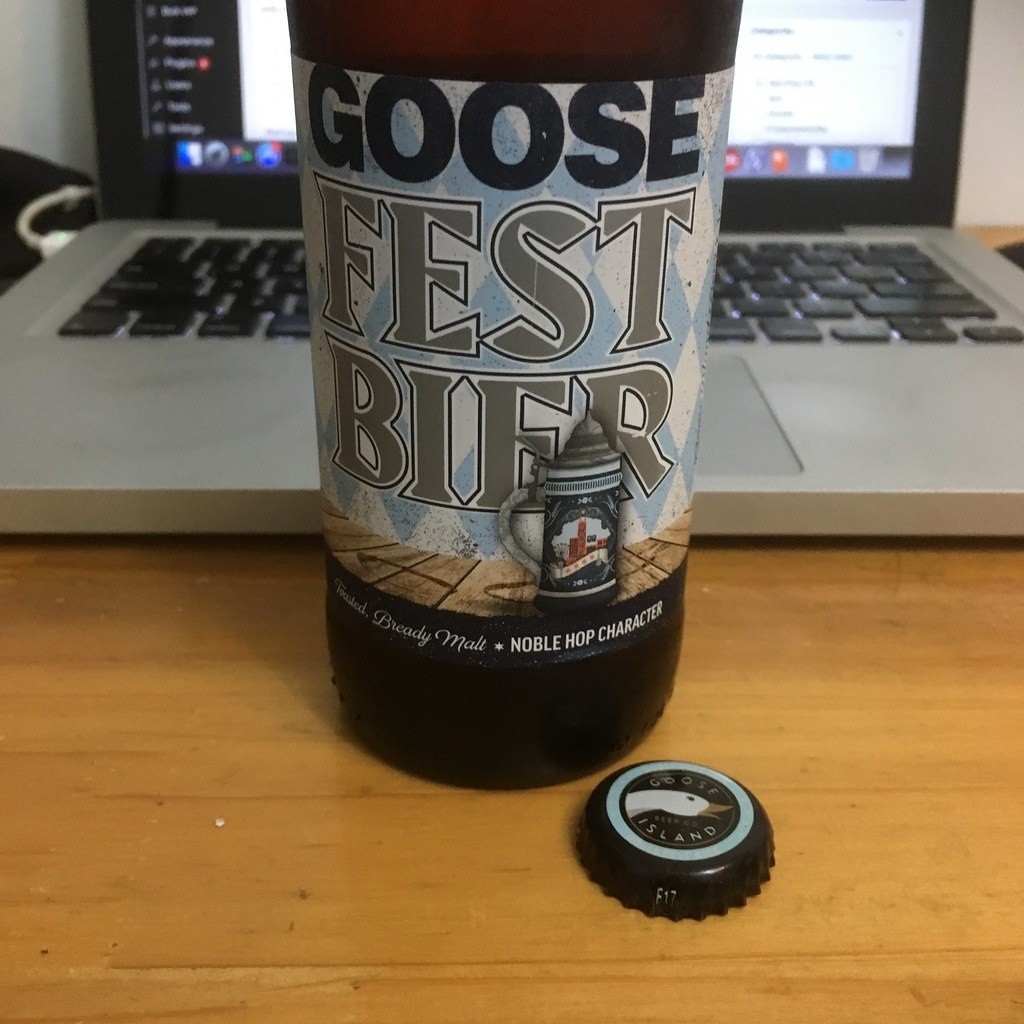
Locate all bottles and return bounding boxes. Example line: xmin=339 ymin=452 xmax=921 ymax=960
xmin=290 ymin=0 xmax=746 ymax=789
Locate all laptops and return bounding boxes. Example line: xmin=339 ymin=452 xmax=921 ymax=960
xmin=0 ymin=0 xmax=1024 ymax=538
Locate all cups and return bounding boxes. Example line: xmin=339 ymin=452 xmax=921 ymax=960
xmin=500 ymin=459 xmax=622 ymax=611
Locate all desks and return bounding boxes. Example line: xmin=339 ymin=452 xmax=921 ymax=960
xmin=0 ymin=225 xmax=1024 ymax=1024
